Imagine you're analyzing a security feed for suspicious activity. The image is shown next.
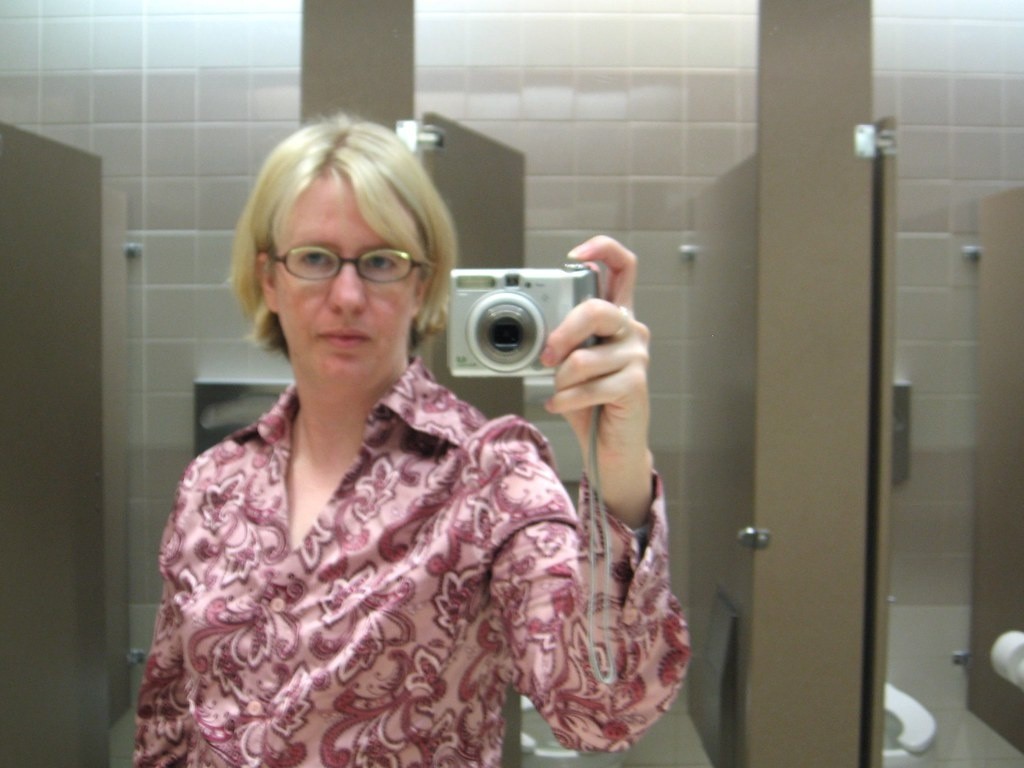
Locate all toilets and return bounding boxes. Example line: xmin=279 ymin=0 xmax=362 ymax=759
xmin=520 ymin=692 xmax=623 ymax=768
xmin=881 ymin=682 xmax=936 ymax=768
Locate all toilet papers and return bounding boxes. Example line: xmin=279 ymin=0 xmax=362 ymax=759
xmin=990 ymin=630 xmax=1024 ymax=685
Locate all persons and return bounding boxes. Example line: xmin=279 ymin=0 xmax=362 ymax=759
xmin=134 ymin=109 xmax=690 ymax=768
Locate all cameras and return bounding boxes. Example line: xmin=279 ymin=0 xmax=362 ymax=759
xmin=445 ymin=262 xmax=600 ymax=383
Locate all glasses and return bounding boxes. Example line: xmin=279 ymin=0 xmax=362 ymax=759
xmin=274 ymin=245 xmax=438 ymax=283
